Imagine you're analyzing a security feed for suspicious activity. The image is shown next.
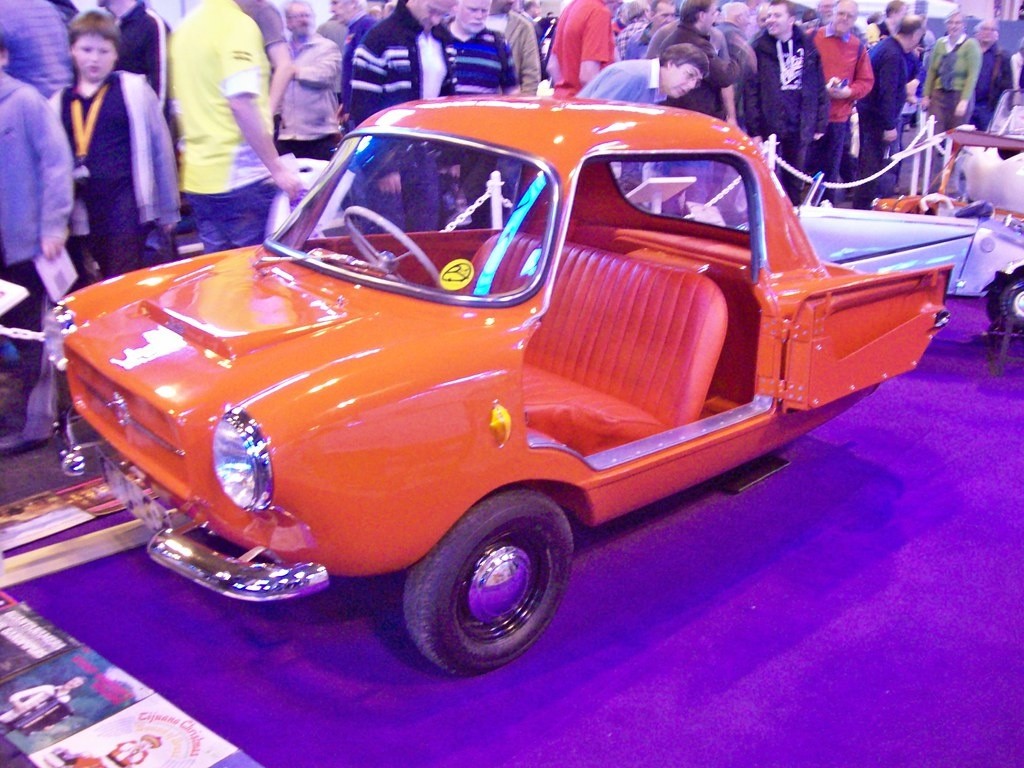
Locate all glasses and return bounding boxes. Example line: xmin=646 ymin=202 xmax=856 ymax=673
xmin=946 ymin=20 xmax=962 ymax=25
xmin=286 ymin=12 xmax=315 ymax=20
xmin=830 ymin=11 xmax=856 ymax=20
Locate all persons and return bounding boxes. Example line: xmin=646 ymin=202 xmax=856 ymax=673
xmin=0 ymin=34 xmax=78 ymax=455
xmin=1 ymin=0 xmax=1023 ymax=119
xmin=743 ymin=0 xmax=830 ymax=207
xmin=427 ymin=0 xmax=522 ymax=232
xmin=914 ymin=8 xmax=984 ymax=194
xmin=802 ymin=0 xmax=876 ymax=208
xmin=47 ymin=5 xmax=182 ymax=284
xmin=850 ymin=13 xmax=928 ymax=212
xmin=346 ymin=0 xmax=463 ymax=236
xmin=273 ymin=0 xmax=344 ymax=162
xmin=166 ymin=0 xmax=311 ymax=256
xmin=967 ymin=17 xmax=1015 ymax=131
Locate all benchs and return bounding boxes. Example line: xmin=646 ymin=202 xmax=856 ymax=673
xmin=446 ymin=233 xmax=733 ymax=458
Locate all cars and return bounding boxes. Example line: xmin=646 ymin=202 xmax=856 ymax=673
xmin=42 ymin=91 xmax=954 ymax=677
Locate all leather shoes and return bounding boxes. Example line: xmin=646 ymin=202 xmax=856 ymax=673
xmin=0 ymin=428 xmax=47 ymax=455
